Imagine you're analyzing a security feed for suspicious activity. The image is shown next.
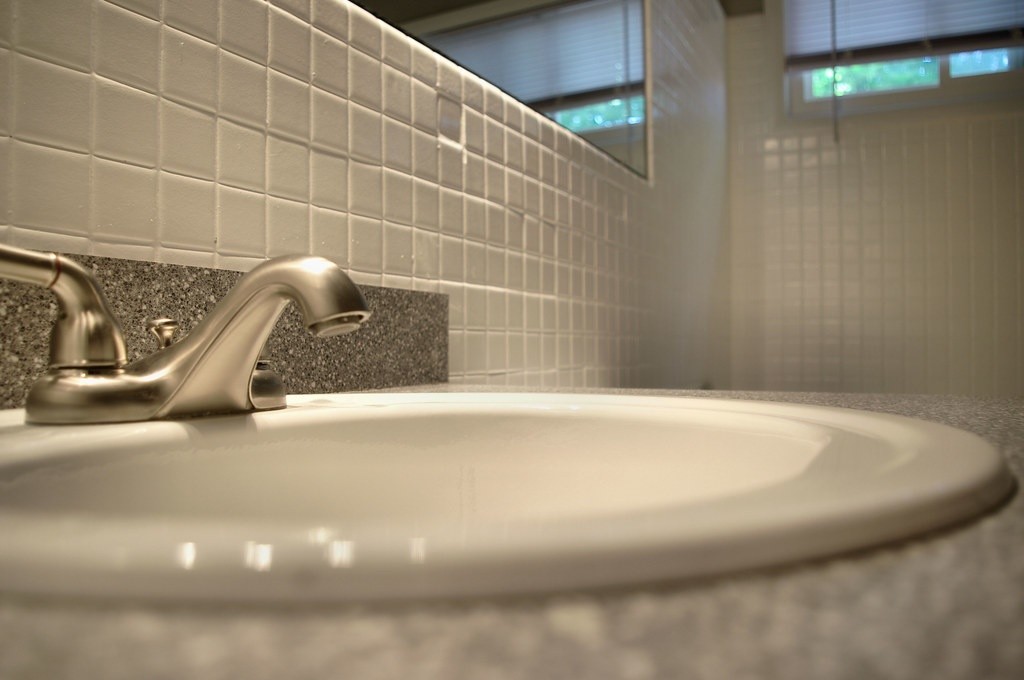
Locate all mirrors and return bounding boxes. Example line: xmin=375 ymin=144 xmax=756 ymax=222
xmin=350 ymin=1 xmax=650 ymax=181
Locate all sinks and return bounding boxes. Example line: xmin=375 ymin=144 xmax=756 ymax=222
xmin=0 ymin=392 xmax=1014 ymax=601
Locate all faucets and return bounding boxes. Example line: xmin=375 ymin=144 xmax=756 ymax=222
xmin=131 ymin=252 xmax=372 ymax=417
